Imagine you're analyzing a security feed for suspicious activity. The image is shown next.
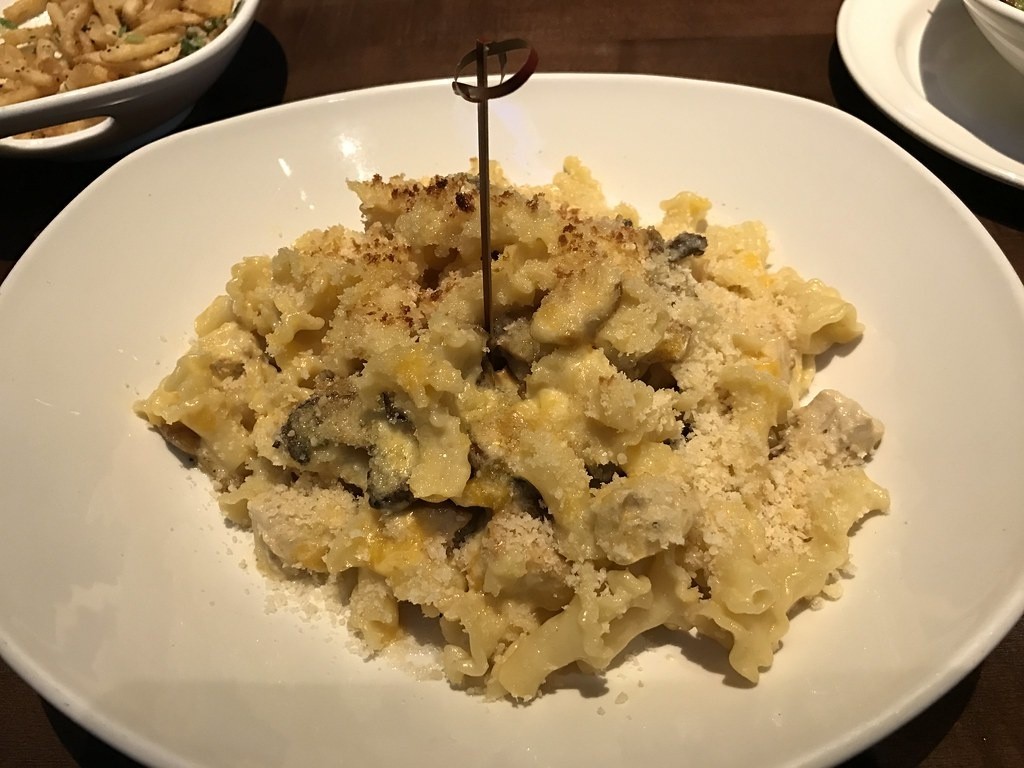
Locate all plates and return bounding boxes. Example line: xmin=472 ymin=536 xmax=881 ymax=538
xmin=836 ymin=0 xmax=1024 ymax=188
xmin=0 ymin=72 xmax=1024 ymax=768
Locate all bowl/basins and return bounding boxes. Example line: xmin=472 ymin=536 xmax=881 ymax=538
xmin=961 ymin=0 xmax=1024 ymax=75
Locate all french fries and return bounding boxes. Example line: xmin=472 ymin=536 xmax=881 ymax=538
xmin=0 ymin=0 xmax=232 ymax=139
